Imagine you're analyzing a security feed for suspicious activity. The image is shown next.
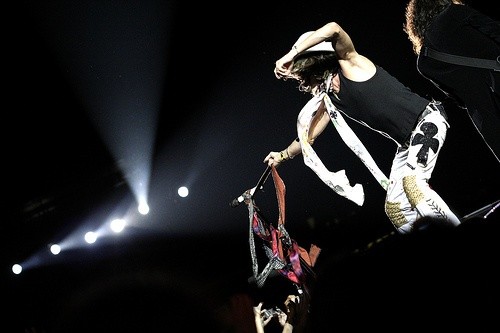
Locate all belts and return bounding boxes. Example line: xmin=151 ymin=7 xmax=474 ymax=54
xmin=401 ymin=103 xmax=437 ymax=149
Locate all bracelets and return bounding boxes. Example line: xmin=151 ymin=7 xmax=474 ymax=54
xmin=293 ymin=45 xmax=301 ymax=56
xmin=287 ymin=148 xmax=295 ymax=160
xmin=280 ymin=150 xmax=287 ymax=162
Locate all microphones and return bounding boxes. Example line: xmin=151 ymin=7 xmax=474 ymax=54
xmin=249 ymin=158 xmax=274 ymax=199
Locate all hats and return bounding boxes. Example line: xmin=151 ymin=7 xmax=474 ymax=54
xmin=284 ymin=30 xmax=335 ymax=52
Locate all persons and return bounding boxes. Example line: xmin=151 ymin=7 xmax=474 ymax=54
xmin=263 ymin=20 xmax=462 ymax=233
xmin=400 ymin=1 xmax=500 ymax=203
